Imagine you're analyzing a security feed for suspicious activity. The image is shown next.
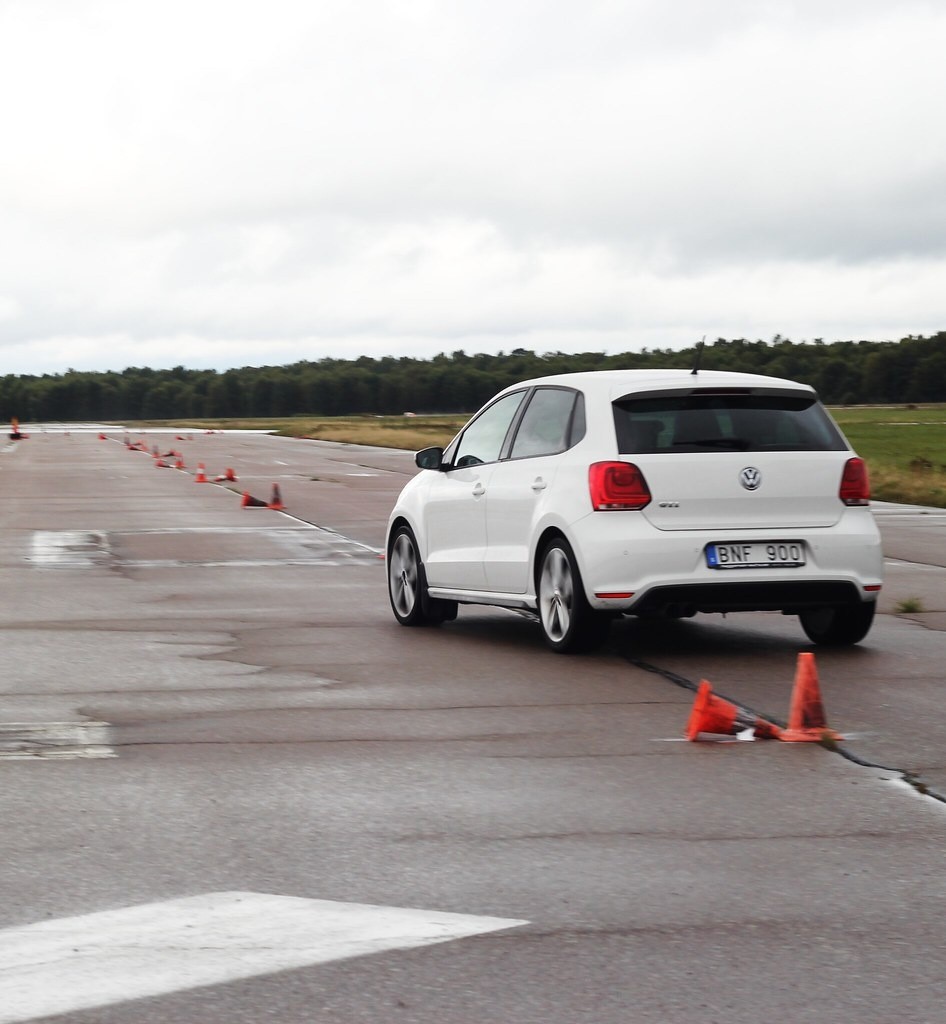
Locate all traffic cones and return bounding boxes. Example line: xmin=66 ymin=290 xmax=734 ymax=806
xmin=98 ymin=434 xmax=108 ymax=441
xmin=780 ymin=652 xmax=860 ymax=746
xmin=683 ymin=678 xmax=787 ymax=741
xmin=213 ymin=468 xmax=239 ymax=481
xmin=175 ymin=459 xmax=186 ymax=469
xmin=127 ymin=440 xmax=183 ymax=457
xmin=156 ymin=460 xmax=173 ymax=468
xmin=176 ymin=436 xmax=186 ymax=440
xmin=242 ymin=491 xmax=268 ymax=509
xmin=194 ymin=463 xmax=208 ymax=482
xmin=268 ymin=483 xmax=288 ymax=510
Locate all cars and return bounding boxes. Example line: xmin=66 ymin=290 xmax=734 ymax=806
xmin=383 ymin=367 xmax=884 ymax=654
